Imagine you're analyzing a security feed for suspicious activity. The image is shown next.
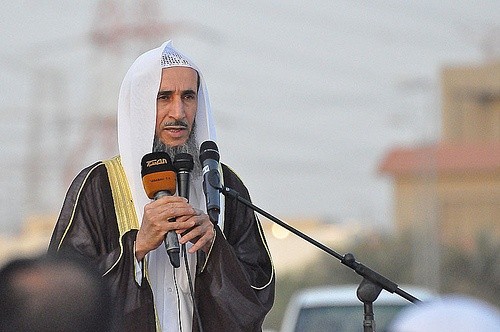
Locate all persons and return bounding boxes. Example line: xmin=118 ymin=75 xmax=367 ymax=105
xmin=44 ymin=39 xmax=276 ymax=332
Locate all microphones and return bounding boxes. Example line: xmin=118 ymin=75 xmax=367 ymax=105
xmin=141 ymin=151 xmax=181 ymax=268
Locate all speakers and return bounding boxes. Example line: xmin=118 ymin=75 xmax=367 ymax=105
xmin=199 ymin=140 xmax=221 ymax=224
xmin=173 ymin=152 xmax=194 ymax=203
